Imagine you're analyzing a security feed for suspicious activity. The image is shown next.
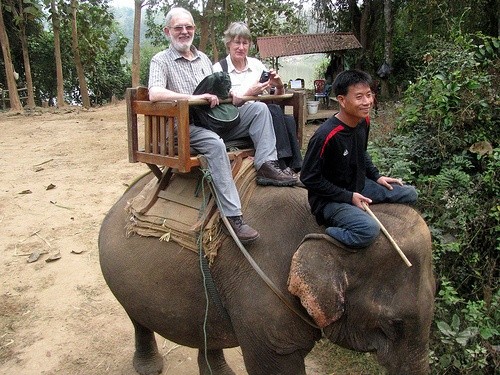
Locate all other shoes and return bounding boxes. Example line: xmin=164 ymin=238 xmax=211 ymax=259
xmin=221 ymin=215 xmax=260 ymax=242
xmin=255 ymin=160 xmax=297 ymax=187
xmin=283 ymin=167 xmax=305 ymax=187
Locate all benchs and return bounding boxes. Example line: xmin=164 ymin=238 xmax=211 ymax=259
xmin=126 ymin=86 xmax=306 ymax=173
xmin=329 ymin=97 xmax=340 ymax=111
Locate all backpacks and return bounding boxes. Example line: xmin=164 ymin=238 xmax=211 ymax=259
xmin=188 ymin=71 xmax=241 ymax=135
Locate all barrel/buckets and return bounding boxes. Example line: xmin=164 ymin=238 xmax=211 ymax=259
xmin=306 ymin=101 xmax=320 ymax=115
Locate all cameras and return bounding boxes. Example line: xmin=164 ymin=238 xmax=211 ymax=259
xmin=259 ymin=69 xmax=270 ymax=82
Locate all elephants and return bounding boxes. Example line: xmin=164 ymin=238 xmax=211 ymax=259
xmin=98 ymin=156 xmax=436 ymax=375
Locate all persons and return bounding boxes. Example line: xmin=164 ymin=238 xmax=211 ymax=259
xmin=148 ymin=6 xmax=299 ymax=242
xmin=212 ymin=21 xmax=306 ymax=188
xmin=299 ymin=69 xmax=418 ymax=248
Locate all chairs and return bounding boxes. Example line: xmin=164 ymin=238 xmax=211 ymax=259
xmin=314 ymin=80 xmax=329 ymax=106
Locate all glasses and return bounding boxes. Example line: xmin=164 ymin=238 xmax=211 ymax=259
xmin=166 ymin=24 xmax=195 ymax=31
